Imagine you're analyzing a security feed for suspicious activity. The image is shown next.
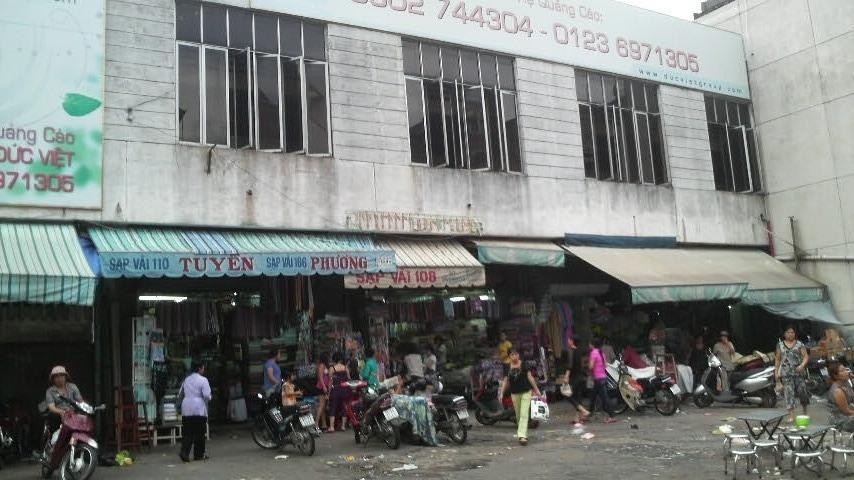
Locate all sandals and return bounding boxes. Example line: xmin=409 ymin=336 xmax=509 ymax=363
xmin=569 ymin=411 xmax=617 ymax=425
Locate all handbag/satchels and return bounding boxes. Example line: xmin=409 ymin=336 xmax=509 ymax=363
xmin=530 ymin=394 xmax=550 ymax=423
xmin=175 ymin=387 xmax=185 ymax=414
xmin=708 ymin=353 xmax=721 ymax=368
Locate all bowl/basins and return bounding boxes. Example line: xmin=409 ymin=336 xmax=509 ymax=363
xmin=795 ymin=415 xmax=812 ymax=430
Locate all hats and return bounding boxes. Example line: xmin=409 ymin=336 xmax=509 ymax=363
xmin=50 ymin=365 xmax=69 ymax=380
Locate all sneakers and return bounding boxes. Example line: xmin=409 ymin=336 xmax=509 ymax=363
xmin=179 ymin=452 xmax=190 ymax=462
xmin=194 ymin=455 xmax=209 ymax=460
xmin=519 ymin=437 xmax=528 ymax=446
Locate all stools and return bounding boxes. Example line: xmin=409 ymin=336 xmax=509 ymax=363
xmin=709 ymin=399 xmax=854 ymax=480
xmin=138 ymin=420 xmax=211 ymax=449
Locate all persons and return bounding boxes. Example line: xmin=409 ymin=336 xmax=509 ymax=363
xmin=176 ymin=360 xmax=213 ymax=461
xmin=587 ymin=329 xmax=736 ymax=423
xmin=311 ymin=330 xmax=543 ymax=444
xmin=263 ymin=348 xmax=282 ymax=400
xmin=825 ymin=362 xmax=854 ymax=432
xmin=560 ymin=334 xmax=591 ymax=424
xmin=774 ymin=324 xmax=809 ymax=423
xmin=280 ymin=371 xmax=303 ymax=407
xmin=44 ymin=365 xmax=85 ymax=446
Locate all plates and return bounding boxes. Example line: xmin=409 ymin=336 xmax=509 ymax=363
xmin=780 ymin=427 xmax=788 ymax=432
xmin=789 ymin=429 xmax=799 ymax=433
xmin=798 ymin=428 xmax=804 ymax=432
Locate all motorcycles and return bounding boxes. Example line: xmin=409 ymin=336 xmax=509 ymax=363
xmin=395 ymin=368 xmax=476 ymax=446
xmin=325 ymin=357 xmax=406 ymax=452
xmin=600 ymin=348 xmax=682 ymax=415
xmin=472 ymin=360 xmax=550 ymax=430
xmin=33 ymin=394 xmax=109 ymax=480
xmin=250 ymin=373 xmax=322 ymax=459
xmin=692 ymin=346 xmax=780 ymax=409
xmin=795 ymin=333 xmax=853 ymax=400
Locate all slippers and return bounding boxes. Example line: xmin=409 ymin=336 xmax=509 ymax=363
xmin=320 ymin=426 xmax=347 ymax=433
